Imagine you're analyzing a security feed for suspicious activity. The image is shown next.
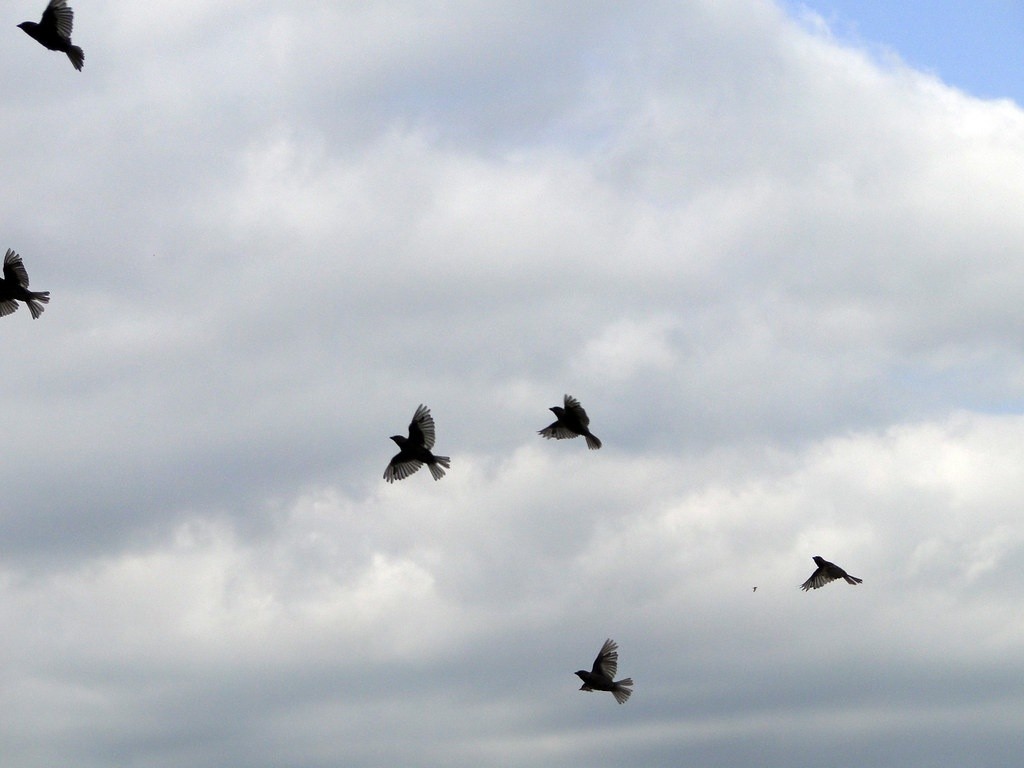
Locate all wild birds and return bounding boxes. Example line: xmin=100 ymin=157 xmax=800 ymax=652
xmin=753 ymin=586 xmax=758 ymax=592
xmin=794 ymin=555 xmax=863 ymax=593
xmin=0 ymin=247 xmax=51 ymax=321
xmin=16 ymin=0 xmax=85 ymax=73
xmin=383 ymin=403 xmax=451 ymax=484
xmin=536 ymin=394 xmax=602 ymax=451
xmin=573 ymin=638 xmax=634 ymax=705
